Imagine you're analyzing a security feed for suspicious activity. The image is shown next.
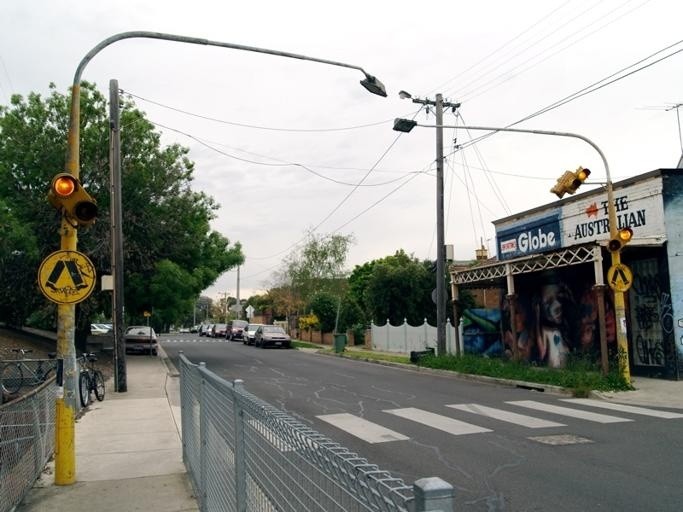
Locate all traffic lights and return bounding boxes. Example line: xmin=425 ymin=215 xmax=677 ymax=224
xmin=50 ymin=173 xmax=98 ymax=227
xmin=607 ymin=224 xmax=632 ymax=253
xmin=564 ymin=167 xmax=591 ymax=196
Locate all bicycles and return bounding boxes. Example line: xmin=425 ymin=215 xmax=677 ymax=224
xmin=0 ymin=345 xmax=57 ymax=395
xmin=77 ymin=350 xmax=105 ymax=408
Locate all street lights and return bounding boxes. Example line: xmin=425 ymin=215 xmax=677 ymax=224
xmin=389 ymin=117 xmax=635 ymax=387
xmin=397 ymin=88 xmax=447 ymax=358
xmin=49 ymin=32 xmax=387 ymax=490
xmin=217 ymin=291 xmax=227 ymax=316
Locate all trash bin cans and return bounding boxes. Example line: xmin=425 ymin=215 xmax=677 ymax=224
xmin=334 ymin=334 xmax=347 ymax=353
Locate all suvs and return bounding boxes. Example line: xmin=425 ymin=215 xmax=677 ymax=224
xmin=225 ymin=319 xmax=248 ymax=341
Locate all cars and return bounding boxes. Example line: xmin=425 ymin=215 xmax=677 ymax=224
xmin=253 ymin=324 xmax=291 ymax=350
xmin=125 ymin=325 xmax=157 ymax=355
xmin=179 ymin=322 xmax=227 ymax=338
xmin=88 ymin=323 xmax=113 ymax=335
xmin=241 ymin=324 xmax=263 ymax=344
